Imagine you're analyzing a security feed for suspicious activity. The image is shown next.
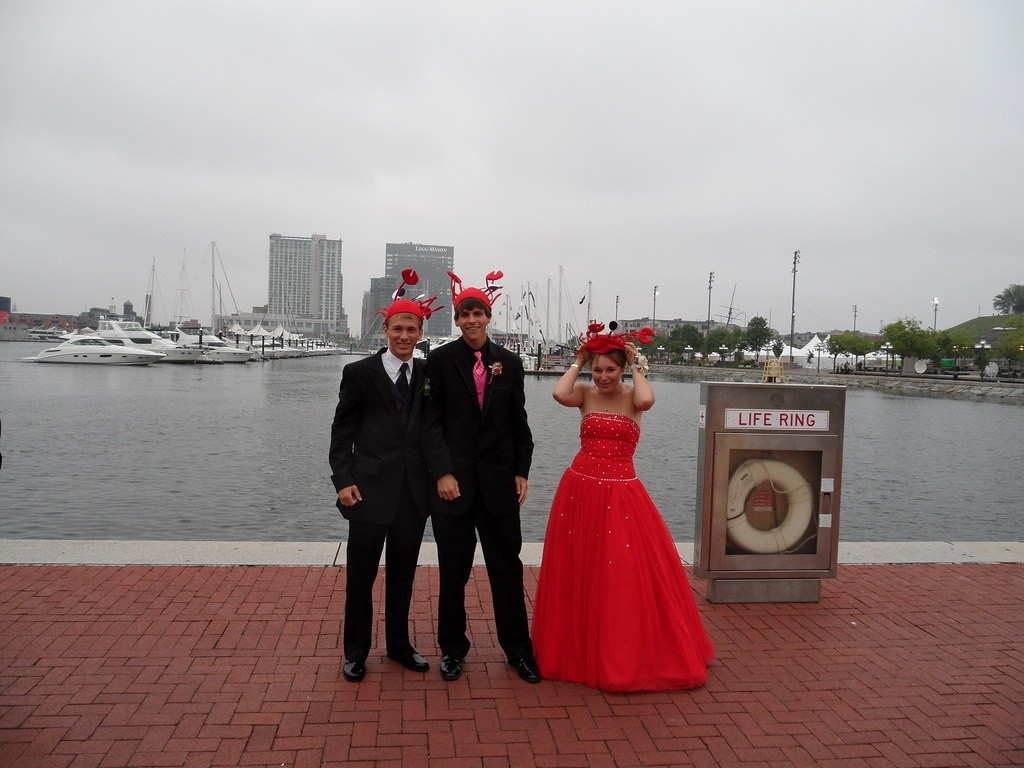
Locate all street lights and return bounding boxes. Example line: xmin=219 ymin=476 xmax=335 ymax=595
xmin=652 ymin=286 xmax=659 ymax=330
xmin=707 ymin=272 xmax=716 ymax=334
xmin=615 ymin=295 xmax=621 ymax=322
xmin=789 ymin=250 xmax=799 ymax=361
xmin=852 ymin=305 xmax=862 ymax=330
xmin=932 ymin=297 xmax=940 ymax=332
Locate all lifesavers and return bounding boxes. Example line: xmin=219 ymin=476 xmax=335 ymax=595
xmin=728 ymin=458 xmax=815 ymax=553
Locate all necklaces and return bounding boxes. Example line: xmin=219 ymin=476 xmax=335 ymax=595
xmin=604 ymin=406 xmax=608 ymax=411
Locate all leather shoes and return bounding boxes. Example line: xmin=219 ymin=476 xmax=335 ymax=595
xmin=507 ymin=656 xmax=540 ymax=683
xmin=342 ymin=659 xmax=366 ymax=682
xmin=387 ymin=647 xmax=429 ymax=672
xmin=440 ymin=654 xmax=464 ymax=681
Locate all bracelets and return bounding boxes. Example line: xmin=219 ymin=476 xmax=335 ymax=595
xmin=630 ymin=355 xmax=649 ymax=378
xmin=571 ymin=364 xmax=581 ymax=371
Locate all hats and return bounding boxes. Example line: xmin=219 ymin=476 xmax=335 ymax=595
xmin=375 ymin=267 xmax=445 ymax=321
xmin=445 ymin=270 xmax=504 ymax=311
xmin=576 ymin=321 xmax=657 ymax=353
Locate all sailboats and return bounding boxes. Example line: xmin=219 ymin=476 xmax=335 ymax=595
xmin=22 ymin=240 xmax=309 ymax=365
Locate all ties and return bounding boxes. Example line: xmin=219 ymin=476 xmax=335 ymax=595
xmin=396 ymin=362 xmax=409 ymax=400
xmin=472 ymin=351 xmax=485 ymax=378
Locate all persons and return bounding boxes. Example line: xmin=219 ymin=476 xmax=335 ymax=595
xmin=755 ymin=360 xmax=759 ymax=370
xmin=531 ymin=334 xmax=716 ymax=691
xmin=425 ymin=287 xmax=541 ymax=683
xmin=857 ymin=361 xmax=863 ymax=371
xmin=836 ymin=360 xmax=854 ymax=375
xmin=329 ymin=300 xmax=431 ymax=683
